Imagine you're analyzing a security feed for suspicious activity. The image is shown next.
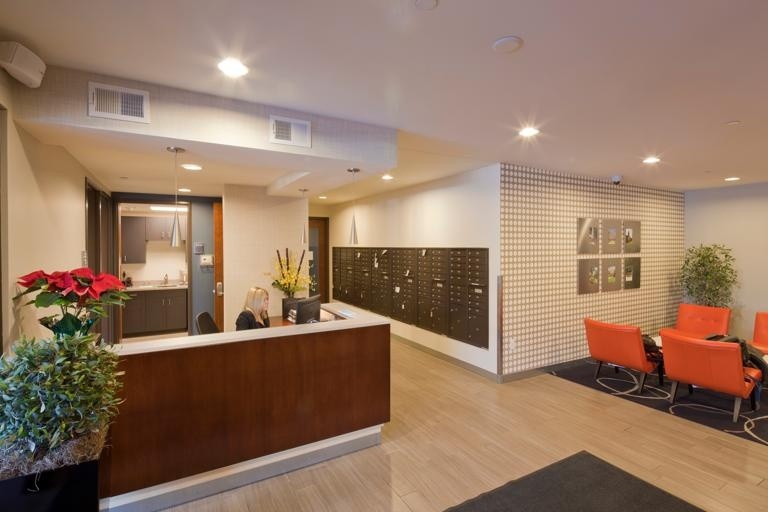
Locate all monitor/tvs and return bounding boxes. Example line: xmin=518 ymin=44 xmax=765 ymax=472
xmin=296 ymin=294 xmax=321 ymax=325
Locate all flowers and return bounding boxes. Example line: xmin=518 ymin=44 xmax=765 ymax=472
xmin=262 ymin=246 xmax=318 ymax=298
xmin=12 ymin=267 xmax=135 ymax=341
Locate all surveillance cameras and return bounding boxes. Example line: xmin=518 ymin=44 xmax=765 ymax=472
xmin=612 ymin=178 xmax=620 ymax=185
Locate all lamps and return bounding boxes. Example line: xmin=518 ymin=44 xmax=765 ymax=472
xmin=166 ymin=147 xmax=187 ymax=248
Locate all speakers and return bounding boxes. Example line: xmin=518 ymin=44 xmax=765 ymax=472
xmin=0 ymin=40 xmax=47 ymax=88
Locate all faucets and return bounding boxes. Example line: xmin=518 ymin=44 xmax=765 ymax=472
xmin=164 ymin=272 xmax=169 ymax=285
xmin=156 ymin=285 xmax=178 ymax=288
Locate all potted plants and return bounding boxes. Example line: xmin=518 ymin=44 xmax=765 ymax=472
xmin=0 ymin=332 xmax=127 ymax=510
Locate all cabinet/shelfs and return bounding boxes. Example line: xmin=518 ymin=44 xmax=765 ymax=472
xmin=123 ymin=292 xmax=145 ymax=335
xmin=146 ymin=290 xmax=188 ymax=333
xmin=121 ymin=217 xmax=174 ymax=264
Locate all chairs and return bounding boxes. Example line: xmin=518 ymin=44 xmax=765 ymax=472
xmin=192 ymin=311 xmax=221 ymax=336
xmin=582 ymin=301 xmax=768 ymax=424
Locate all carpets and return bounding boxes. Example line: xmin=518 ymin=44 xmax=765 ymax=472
xmin=443 ymin=447 xmax=711 ymax=512
xmin=537 ymin=353 xmax=767 ymax=447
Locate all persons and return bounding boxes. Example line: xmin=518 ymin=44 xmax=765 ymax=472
xmin=234 ymin=285 xmax=270 ymax=331
